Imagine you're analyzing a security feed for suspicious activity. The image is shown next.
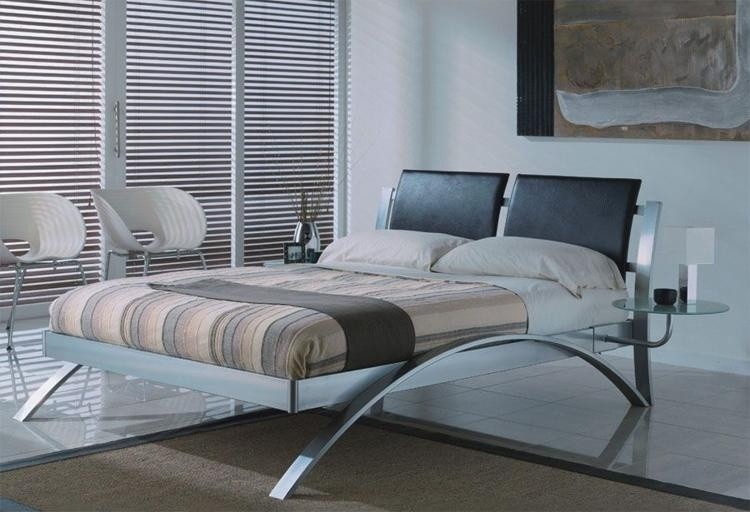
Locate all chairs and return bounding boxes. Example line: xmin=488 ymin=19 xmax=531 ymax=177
xmin=0 ymin=193 xmax=88 ymax=350
xmin=90 ymin=188 xmax=207 ymax=280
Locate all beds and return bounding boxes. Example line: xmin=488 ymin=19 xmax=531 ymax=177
xmin=14 ymin=170 xmax=662 ymax=501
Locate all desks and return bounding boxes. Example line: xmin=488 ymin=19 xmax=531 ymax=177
xmin=604 ymin=298 xmax=730 ymax=346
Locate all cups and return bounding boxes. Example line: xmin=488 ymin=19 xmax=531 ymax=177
xmin=310 ymin=252 xmax=323 ymax=263
xmin=653 ymin=288 xmax=677 ymax=305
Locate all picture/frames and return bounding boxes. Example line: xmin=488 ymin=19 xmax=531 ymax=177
xmin=284 ymin=240 xmax=305 ymax=263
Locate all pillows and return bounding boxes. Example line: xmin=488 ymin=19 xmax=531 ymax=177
xmin=316 ymin=228 xmax=471 ymax=277
xmin=431 ymin=235 xmax=628 ymax=298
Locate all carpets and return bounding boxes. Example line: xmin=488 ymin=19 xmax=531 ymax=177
xmin=0 ymin=408 xmax=749 ymax=512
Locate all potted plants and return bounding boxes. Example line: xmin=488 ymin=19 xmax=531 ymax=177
xmin=271 ymin=141 xmax=370 ymax=261
